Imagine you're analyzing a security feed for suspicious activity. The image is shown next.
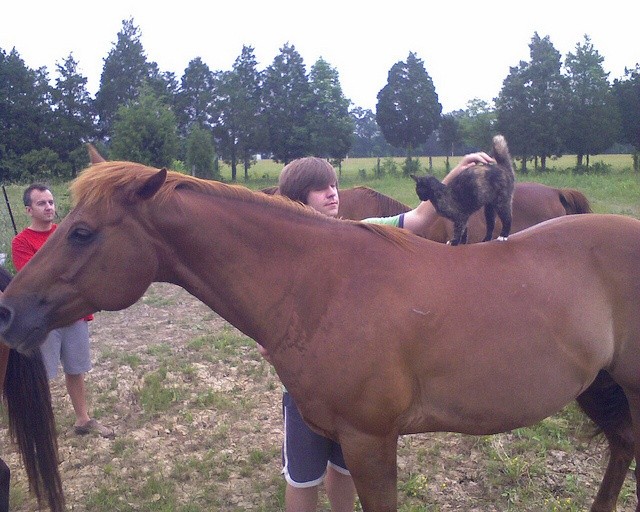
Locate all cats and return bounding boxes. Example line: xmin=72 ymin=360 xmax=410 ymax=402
xmin=409 ymin=133 xmax=516 ymax=246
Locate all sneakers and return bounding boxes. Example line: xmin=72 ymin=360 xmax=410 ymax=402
xmin=73 ymin=418 xmax=114 ymax=437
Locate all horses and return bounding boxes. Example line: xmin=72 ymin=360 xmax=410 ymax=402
xmin=415 ymin=181 xmax=591 ymax=246
xmin=0 ymin=267 xmax=68 ymax=511
xmin=0 ymin=141 xmax=639 ymax=512
xmin=257 ymin=186 xmax=413 ymax=222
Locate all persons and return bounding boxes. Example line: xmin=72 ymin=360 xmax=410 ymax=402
xmin=279 ymin=152 xmax=494 ymax=508
xmin=13 ymin=184 xmax=113 ymax=436
